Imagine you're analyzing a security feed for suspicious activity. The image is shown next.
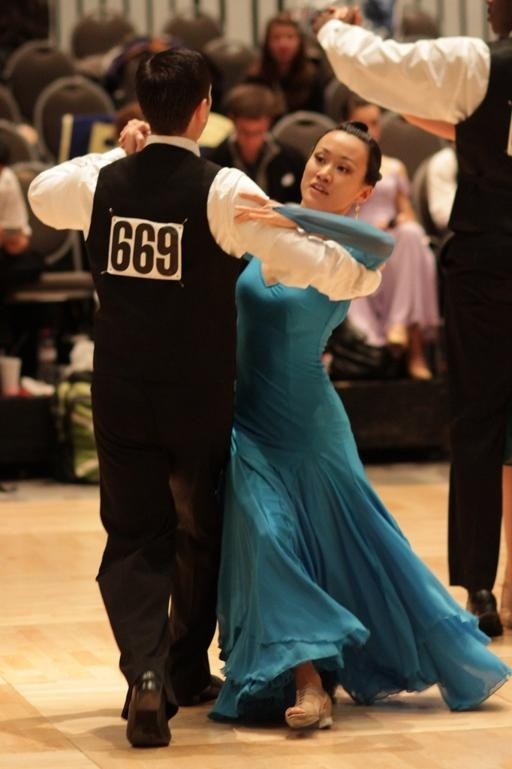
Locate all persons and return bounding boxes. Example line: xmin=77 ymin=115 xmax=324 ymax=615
xmin=118 ymin=118 xmax=398 ymax=733
xmin=23 ymin=43 xmax=386 ymax=750
xmin=1 ymin=0 xmax=460 ymax=395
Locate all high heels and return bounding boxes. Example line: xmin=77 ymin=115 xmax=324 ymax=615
xmin=284 ymin=686 xmax=334 ymax=730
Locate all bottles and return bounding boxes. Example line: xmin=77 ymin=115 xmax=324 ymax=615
xmin=34 ymin=328 xmax=58 ymax=382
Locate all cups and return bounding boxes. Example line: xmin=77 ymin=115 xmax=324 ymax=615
xmin=1 ymin=357 xmax=21 ymax=394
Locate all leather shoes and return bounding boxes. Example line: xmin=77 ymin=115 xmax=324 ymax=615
xmin=177 ymin=674 xmax=225 ymax=708
xmin=466 ymin=588 xmax=503 ymax=638
xmin=126 ymin=669 xmax=172 ymax=748
